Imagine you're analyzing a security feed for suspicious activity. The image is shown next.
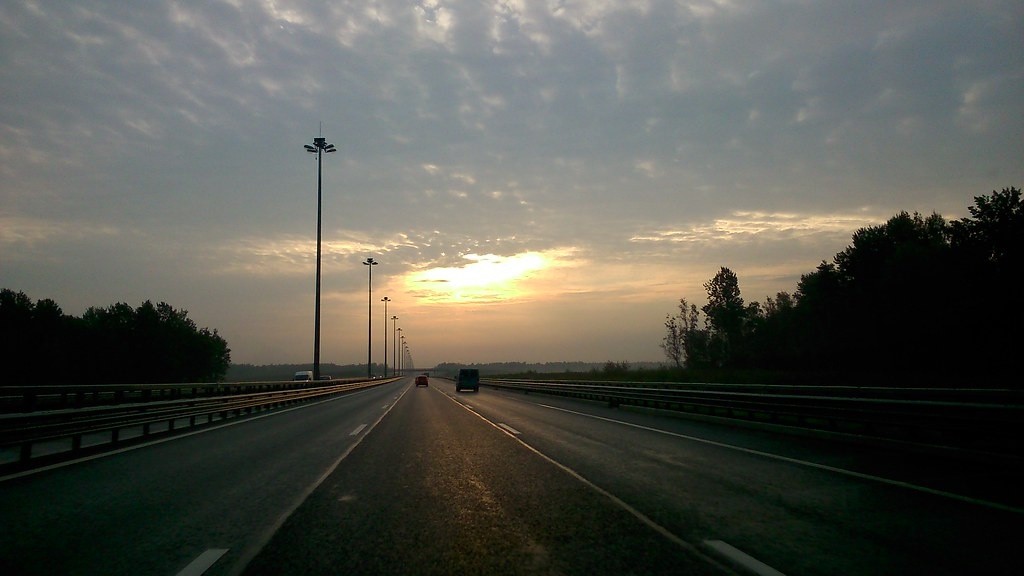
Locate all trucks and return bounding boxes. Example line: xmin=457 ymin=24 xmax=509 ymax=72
xmin=455 ymin=367 xmax=479 ymax=393
xmin=294 ymin=371 xmax=313 ymax=390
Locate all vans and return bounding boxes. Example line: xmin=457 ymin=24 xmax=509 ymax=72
xmin=320 ymin=375 xmax=331 ymax=380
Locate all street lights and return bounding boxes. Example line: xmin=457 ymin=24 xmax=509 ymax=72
xmin=304 ymin=120 xmax=338 ymax=381
xmin=391 ymin=316 xmax=399 ymax=377
xmin=396 ymin=327 xmax=402 ymax=377
xmin=382 ymin=297 xmax=391 ymax=378
xmin=362 ymin=257 xmax=379 ymax=378
xmin=400 ymin=336 xmax=411 ymax=376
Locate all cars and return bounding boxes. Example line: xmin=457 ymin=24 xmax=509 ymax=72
xmin=415 ymin=375 xmax=428 ymax=387
